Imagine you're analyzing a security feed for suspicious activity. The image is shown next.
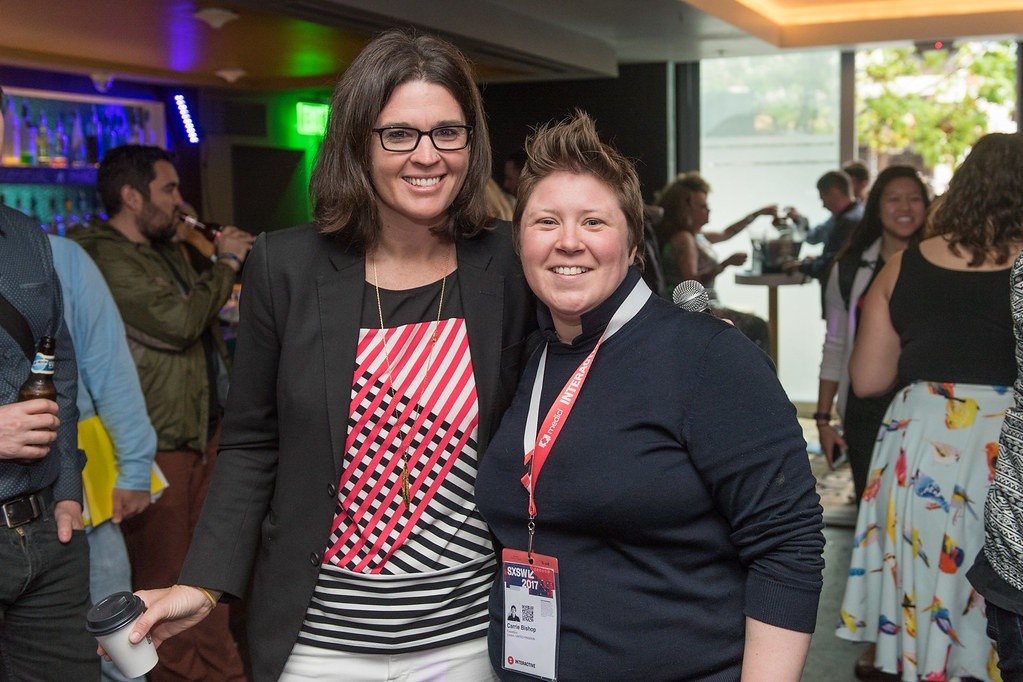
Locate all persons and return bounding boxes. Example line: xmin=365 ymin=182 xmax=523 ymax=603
xmin=484 ymin=152 xmax=525 ymax=221
xmin=644 ymin=133 xmax=1022 ymax=681
xmin=0 ymin=105 xmax=257 ymax=682
xmin=95 ymin=29 xmax=551 ymax=682
xmin=473 ymin=108 xmax=827 ymax=681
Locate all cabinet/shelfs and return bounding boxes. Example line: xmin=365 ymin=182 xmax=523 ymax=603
xmin=0 ymin=63 xmax=199 ymax=244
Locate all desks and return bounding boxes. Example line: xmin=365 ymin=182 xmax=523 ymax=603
xmin=736 ymin=271 xmax=811 ymax=371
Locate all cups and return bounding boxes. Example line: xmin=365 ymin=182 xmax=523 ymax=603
xmin=84 ymin=591 xmax=159 ymax=679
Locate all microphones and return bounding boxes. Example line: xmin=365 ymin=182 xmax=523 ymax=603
xmin=672 ymin=279 xmax=714 ymax=315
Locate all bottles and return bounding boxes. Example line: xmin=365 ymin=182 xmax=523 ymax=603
xmin=178 ymin=210 xmax=253 ymax=256
xmin=10 ymin=336 xmax=55 ymax=466
xmin=1 ymin=96 xmax=151 ymax=237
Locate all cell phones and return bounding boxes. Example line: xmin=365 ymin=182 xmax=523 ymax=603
xmin=830 ymin=442 xmax=849 ymax=471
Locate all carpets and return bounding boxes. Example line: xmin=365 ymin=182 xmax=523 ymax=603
xmin=803 ymin=436 xmax=860 ymax=526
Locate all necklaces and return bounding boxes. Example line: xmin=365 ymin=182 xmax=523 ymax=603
xmin=371 ymin=244 xmax=452 ymax=512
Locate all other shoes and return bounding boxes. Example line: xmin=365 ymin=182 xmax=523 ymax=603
xmin=856 ymin=650 xmax=879 ymax=678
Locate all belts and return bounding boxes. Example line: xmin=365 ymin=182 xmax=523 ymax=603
xmin=1 ymin=489 xmax=52 ymax=529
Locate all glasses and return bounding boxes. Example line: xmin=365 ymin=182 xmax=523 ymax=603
xmin=370 ymin=124 xmax=474 ymax=151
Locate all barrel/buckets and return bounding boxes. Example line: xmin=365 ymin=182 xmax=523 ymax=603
xmin=760 ymin=238 xmax=805 ymax=273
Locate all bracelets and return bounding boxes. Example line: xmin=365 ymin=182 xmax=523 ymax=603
xmin=812 ymin=412 xmax=832 ymax=421
xmin=218 ymin=253 xmax=243 ymax=272
xmin=196 ymin=587 xmax=217 ymax=612
xmin=816 ymin=422 xmax=828 ymax=427
